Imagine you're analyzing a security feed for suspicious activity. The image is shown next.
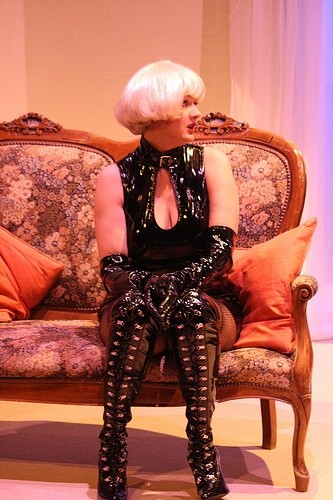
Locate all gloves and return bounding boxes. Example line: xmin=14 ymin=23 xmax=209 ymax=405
xmin=100 ymin=253 xmax=164 ymax=328
xmin=155 ymin=226 xmax=237 ymax=330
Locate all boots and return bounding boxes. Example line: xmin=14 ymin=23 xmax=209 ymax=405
xmin=98 ymin=289 xmax=161 ymax=500
xmin=161 ymin=296 xmax=229 ymax=500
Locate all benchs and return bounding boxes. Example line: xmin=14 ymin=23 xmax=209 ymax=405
xmin=0 ymin=111 xmax=319 ymax=493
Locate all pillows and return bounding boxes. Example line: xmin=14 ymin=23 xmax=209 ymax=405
xmin=226 ymin=217 xmax=317 ymax=355
xmin=0 ymin=224 xmax=64 ymax=323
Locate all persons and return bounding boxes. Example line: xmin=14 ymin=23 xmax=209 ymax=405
xmin=95 ymin=62 xmax=238 ymax=500
